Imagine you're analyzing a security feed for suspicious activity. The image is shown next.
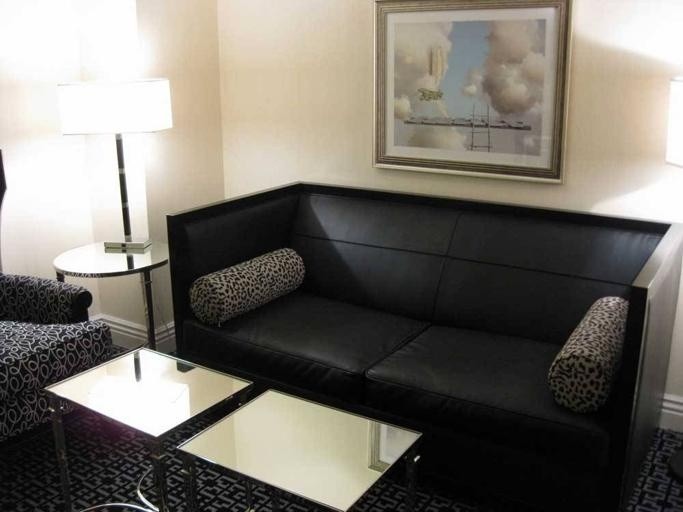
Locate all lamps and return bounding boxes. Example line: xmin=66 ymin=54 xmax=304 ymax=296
xmin=666 ymin=76 xmax=683 ymax=169
xmin=102 ymin=76 xmax=173 ymax=250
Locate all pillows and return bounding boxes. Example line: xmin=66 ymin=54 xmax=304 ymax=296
xmin=546 ymin=296 xmax=628 ymax=413
xmin=191 ymin=249 xmax=304 ymax=326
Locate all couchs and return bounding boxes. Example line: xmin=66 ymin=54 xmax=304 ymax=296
xmin=0 ymin=274 xmax=111 ymax=512
xmin=165 ymin=182 xmax=683 ymax=512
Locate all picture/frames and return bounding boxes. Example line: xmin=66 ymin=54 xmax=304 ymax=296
xmin=373 ymin=1 xmax=571 ymax=184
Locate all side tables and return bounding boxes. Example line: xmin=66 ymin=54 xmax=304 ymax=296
xmin=53 ymin=240 xmax=169 ymax=350
